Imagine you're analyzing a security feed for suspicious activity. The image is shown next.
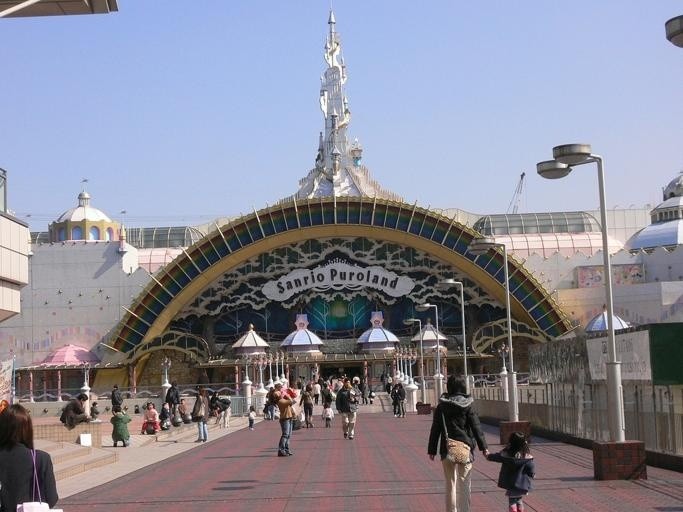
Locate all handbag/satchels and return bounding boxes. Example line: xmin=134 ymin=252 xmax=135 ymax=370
xmin=16 ymin=500 xmax=63 ymax=512
xmin=445 ymin=436 xmax=471 ymax=466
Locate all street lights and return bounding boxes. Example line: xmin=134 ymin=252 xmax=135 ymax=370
xmin=437 ymin=278 xmax=472 ymax=398
xmin=464 ymin=233 xmax=534 ymax=446
xmin=160 ymin=355 xmax=172 ymax=405
xmin=80 ymin=360 xmax=91 ymax=401
xmin=496 ymin=341 xmax=509 ymax=401
xmin=235 ymin=348 xmax=290 ymax=416
xmin=531 ymin=141 xmax=650 ymax=482
xmin=413 ymin=301 xmax=444 ymax=403
xmin=390 ymin=342 xmax=419 ymax=415
xmin=402 ymin=316 xmax=431 ymax=416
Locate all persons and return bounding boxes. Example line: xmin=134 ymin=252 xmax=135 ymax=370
xmin=158 ymin=402 xmax=170 ymax=431
xmin=260 ymin=370 xmax=409 ymax=457
xmin=90 ymin=402 xmax=98 ymax=420
xmin=247 ymin=405 xmax=257 ymax=430
xmin=482 ymin=432 xmax=535 ymax=512
xmin=179 ymin=397 xmax=187 ymax=420
xmin=190 ymin=386 xmax=208 ymax=442
xmin=110 ymin=384 xmax=123 ymax=415
xmin=109 ymin=406 xmax=132 ymax=447
xmin=165 ymin=381 xmax=179 ymax=417
xmin=427 ymin=373 xmax=488 ymax=512
xmin=193 ymin=384 xmax=230 ymax=429
xmin=59 ymin=393 xmax=88 ymax=428
xmin=0 ymin=404 xmax=58 ymax=511
xmin=141 ymin=399 xmax=158 ymax=436
